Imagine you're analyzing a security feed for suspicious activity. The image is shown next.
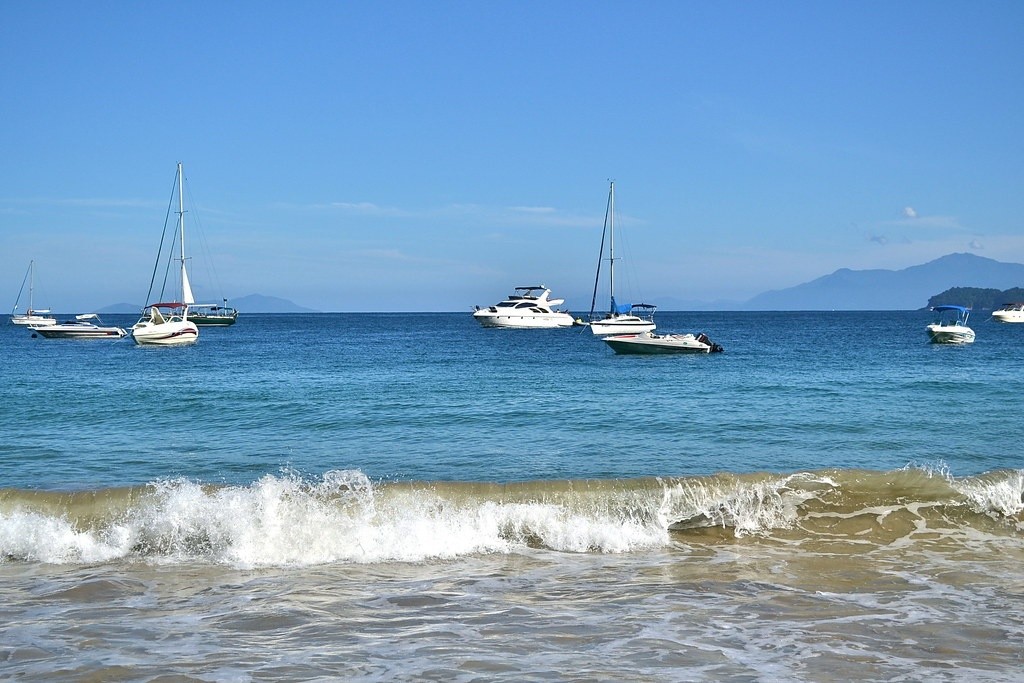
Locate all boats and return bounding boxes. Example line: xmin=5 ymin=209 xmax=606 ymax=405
xmin=35 ymin=314 xmax=128 ymax=340
xmin=992 ymin=302 xmax=1024 ymax=322
xmin=600 ymin=331 xmax=725 ymax=354
xmin=131 ymin=302 xmax=199 ymax=346
xmin=473 ymin=285 xmax=576 ymax=328
xmin=925 ymin=305 xmax=976 ymax=344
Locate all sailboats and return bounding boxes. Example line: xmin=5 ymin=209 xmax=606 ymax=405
xmin=11 ymin=259 xmax=57 ymax=326
xmin=591 ymin=181 xmax=657 ymax=335
xmin=141 ymin=161 xmax=239 ymax=327
